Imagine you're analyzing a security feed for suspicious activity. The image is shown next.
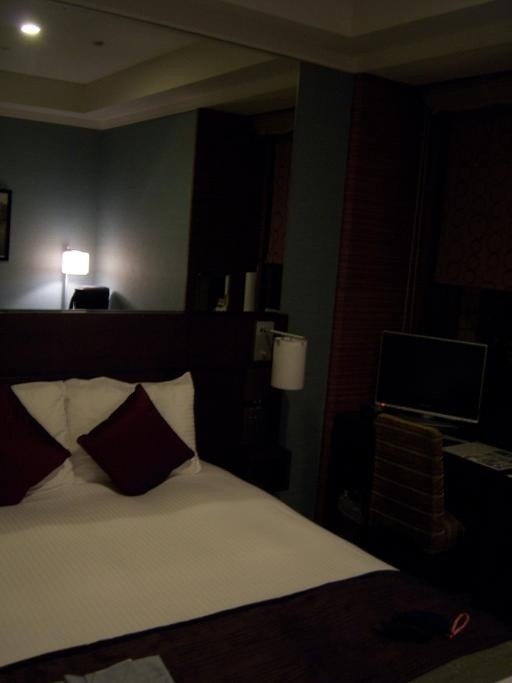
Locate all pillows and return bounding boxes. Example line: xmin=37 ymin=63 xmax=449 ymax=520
xmin=0 ymin=371 xmax=206 ymax=509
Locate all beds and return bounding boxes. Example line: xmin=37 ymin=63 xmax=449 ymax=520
xmin=0 ymin=312 xmax=402 ymax=675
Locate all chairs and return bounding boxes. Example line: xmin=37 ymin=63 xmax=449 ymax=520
xmin=366 ymin=410 xmax=470 ymax=553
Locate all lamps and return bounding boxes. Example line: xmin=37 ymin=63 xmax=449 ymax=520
xmin=253 ymin=317 xmax=310 ymax=393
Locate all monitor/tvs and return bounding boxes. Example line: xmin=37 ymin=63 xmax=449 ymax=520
xmin=373 ymin=329 xmax=487 ymax=429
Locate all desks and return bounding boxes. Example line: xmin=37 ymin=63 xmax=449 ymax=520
xmin=328 ymin=405 xmax=512 ymax=625
